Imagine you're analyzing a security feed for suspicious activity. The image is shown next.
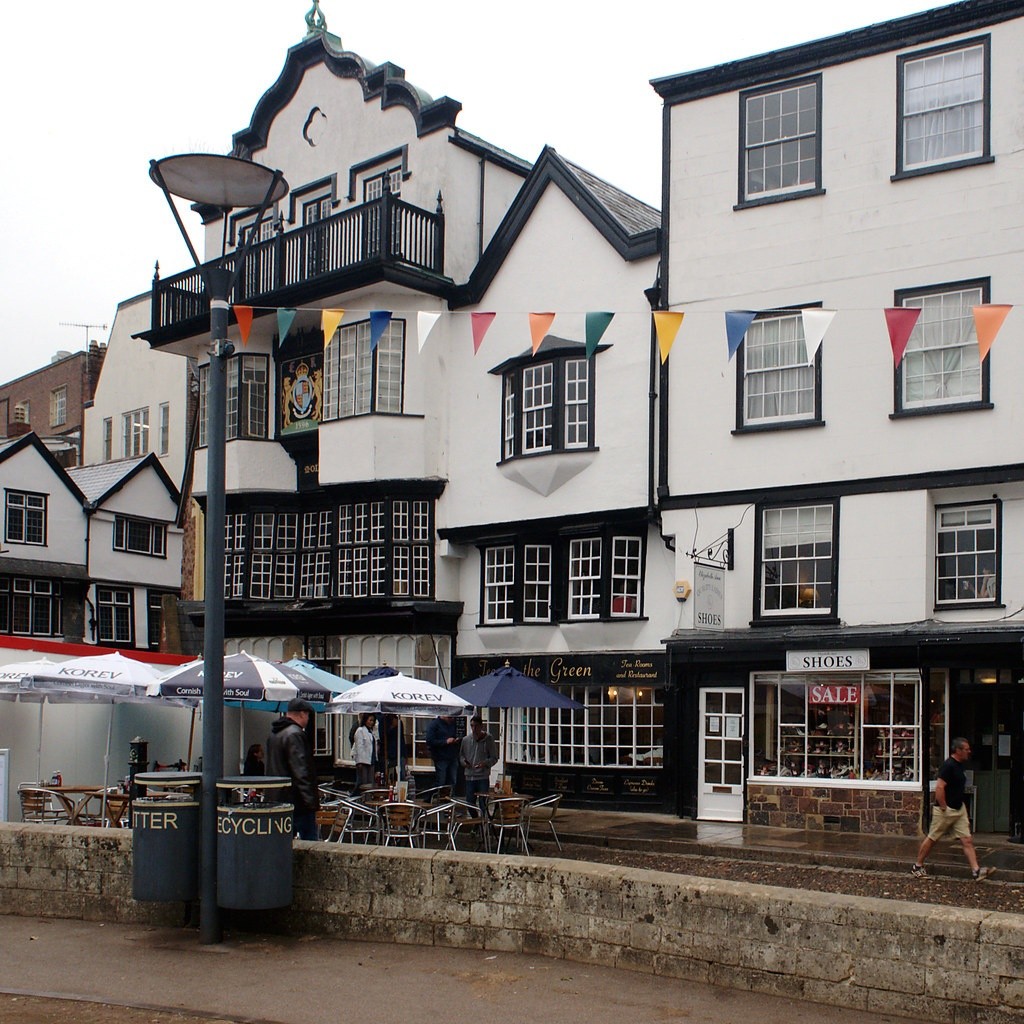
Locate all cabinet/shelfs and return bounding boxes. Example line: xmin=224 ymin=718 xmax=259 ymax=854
xmin=780 ymin=725 xmax=914 ymax=781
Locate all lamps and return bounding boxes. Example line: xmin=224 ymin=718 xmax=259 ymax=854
xmin=801 ymin=588 xmax=821 ymax=608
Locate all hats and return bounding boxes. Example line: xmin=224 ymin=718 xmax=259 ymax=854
xmin=288 ymin=698 xmax=314 ymax=712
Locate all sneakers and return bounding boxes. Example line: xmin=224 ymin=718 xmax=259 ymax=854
xmin=972 ymin=866 xmax=996 ymax=883
xmin=911 ymin=863 xmax=931 ymax=880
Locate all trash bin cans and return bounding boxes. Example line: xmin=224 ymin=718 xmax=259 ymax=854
xmin=132 ymin=772 xmax=202 ymax=902
xmin=215 ymin=775 xmax=296 ymax=908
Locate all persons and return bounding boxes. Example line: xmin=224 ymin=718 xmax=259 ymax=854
xmin=244 ymin=744 xmax=266 ymax=776
xmin=266 ymin=698 xmax=321 ymax=841
xmin=458 ymin=716 xmax=498 ymax=835
xmin=0 ymin=656 xmax=58 ymax=818
xmin=425 ymin=716 xmax=460 ymax=816
xmin=349 ymin=713 xmax=378 ymax=796
xmin=911 ymin=737 xmax=996 ymax=882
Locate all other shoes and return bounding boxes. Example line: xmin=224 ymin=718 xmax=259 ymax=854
xmin=469 ymin=827 xmax=479 ymax=839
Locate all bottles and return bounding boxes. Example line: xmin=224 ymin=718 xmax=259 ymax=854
xmin=51 ymin=771 xmax=62 ymax=787
xmin=376 ymin=771 xmax=385 ymax=789
xmin=388 ymin=785 xmax=398 ymax=802
xmin=495 ymin=779 xmax=502 ymax=795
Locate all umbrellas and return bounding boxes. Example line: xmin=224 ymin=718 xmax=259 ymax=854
xmin=448 ymin=660 xmax=589 ymax=794
xmin=18 ymin=651 xmax=185 ymax=825
xmin=326 ymin=662 xmax=477 ymax=802
xmin=145 ymin=650 xmax=401 ymax=802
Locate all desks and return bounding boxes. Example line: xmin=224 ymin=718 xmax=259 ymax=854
xmin=39 ymin=786 xmax=105 ymax=826
xmin=83 ymin=792 xmax=131 ymax=829
xmin=361 ymin=801 xmax=433 ymax=848
xmin=472 ymin=791 xmax=533 ymax=852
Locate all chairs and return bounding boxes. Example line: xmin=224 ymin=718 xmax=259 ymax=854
xmin=14 ymin=780 xmax=564 ymax=858
